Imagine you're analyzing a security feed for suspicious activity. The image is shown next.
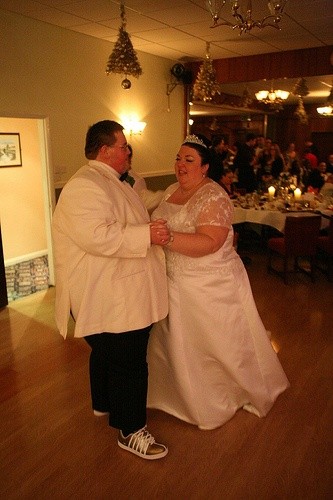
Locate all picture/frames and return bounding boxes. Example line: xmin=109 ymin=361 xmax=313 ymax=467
xmin=0 ymin=132 xmax=22 ymax=169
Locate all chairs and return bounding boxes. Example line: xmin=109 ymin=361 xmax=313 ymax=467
xmin=267 ymin=215 xmax=322 ymax=285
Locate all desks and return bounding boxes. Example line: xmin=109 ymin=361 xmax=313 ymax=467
xmin=232 ymin=206 xmax=333 ymax=235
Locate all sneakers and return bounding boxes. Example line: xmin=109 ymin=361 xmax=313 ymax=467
xmin=118 ymin=425 xmax=169 ymax=459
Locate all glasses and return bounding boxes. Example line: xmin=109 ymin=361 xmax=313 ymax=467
xmin=109 ymin=144 xmax=129 ymax=151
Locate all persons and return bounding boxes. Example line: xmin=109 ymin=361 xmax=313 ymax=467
xmin=207 ymin=132 xmax=333 ymax=199
xmin=51 ymin=119 xmax=169 ymax=459
xmin=146 ymin=134 xmax=289 ymax=428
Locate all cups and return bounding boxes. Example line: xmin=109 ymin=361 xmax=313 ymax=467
xmin=268 ymin=188 xmax=275 ymax=202
xmin=294 ymin=191 xmax=302 ymax=199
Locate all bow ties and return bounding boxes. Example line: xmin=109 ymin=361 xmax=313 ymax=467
xmin=119 ymin=171 xmax=135 ymax=188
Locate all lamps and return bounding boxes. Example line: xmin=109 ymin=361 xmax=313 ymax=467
xmin=204 ymin=0 xmax=287 ymax=36
